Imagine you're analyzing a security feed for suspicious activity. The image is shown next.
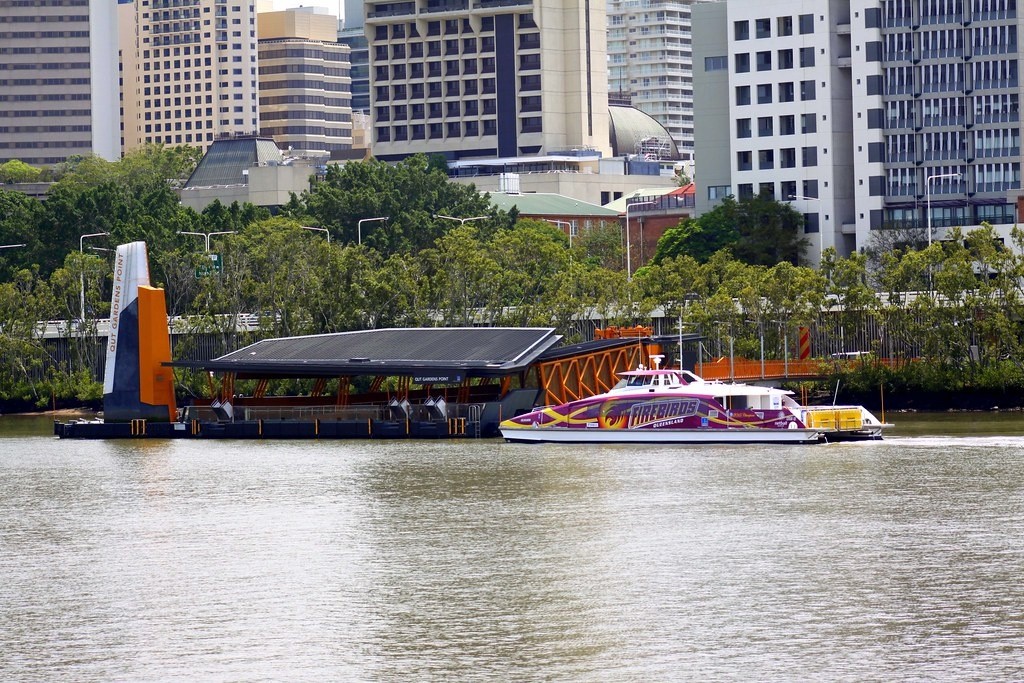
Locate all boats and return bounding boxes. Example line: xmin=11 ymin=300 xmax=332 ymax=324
xmin=498 ymin=319 xmax=895 ymax=444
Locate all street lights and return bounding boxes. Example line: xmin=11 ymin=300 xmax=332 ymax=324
xmin=80 ymin=232 xmax=111 ymax=319
xmin=433 ymin=213 xmax=490 ymax=227
xmin=543 ymin=218 xmax=573 ymax=297
xmin=789 ymin=194 xmax=823 ymax=273
xmin=627 ymin=201 xmax=658 ymax=298
xmin=358 ymin=216 xmax=389 ymax=283
xmin=175 ymin=230 xmax=238 ymax=314
xmin=928 ymin=174 xmax=962 ymax=298
xmin=301 ymin=225 xmax=330 ymax=284
